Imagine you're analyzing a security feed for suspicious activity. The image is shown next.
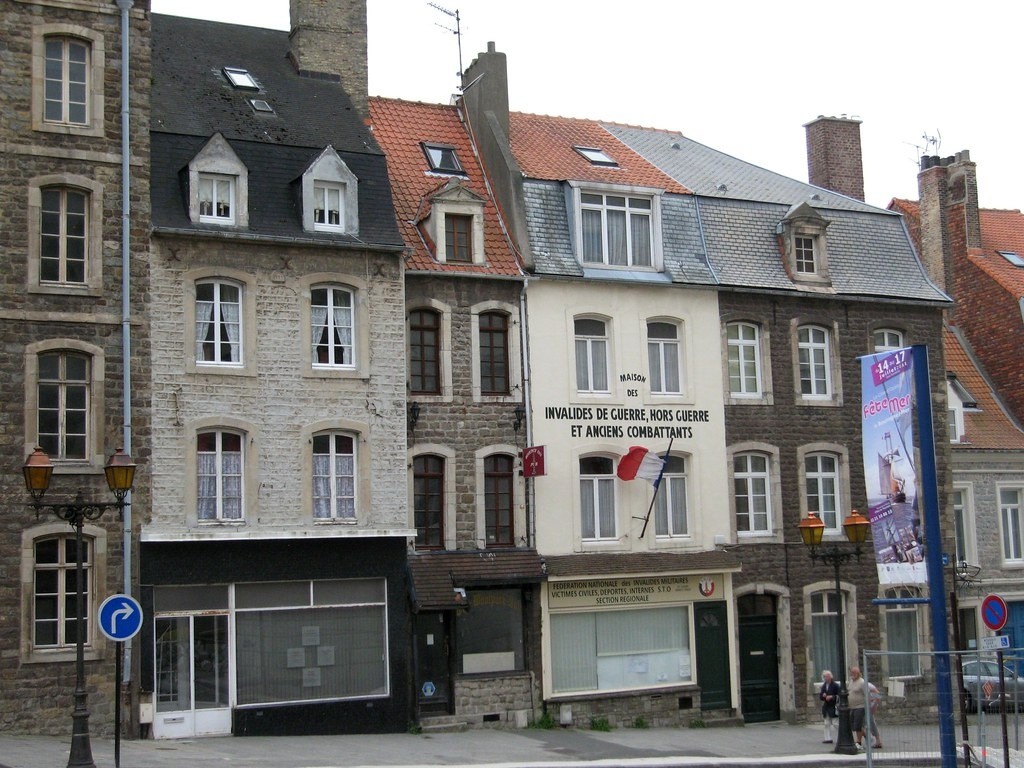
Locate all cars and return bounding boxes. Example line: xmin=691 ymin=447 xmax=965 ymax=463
xmin=961 ymin=661 xmax=1024 ymax=712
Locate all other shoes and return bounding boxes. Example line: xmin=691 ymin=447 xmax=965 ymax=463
xmin=854 ymin=742 xmax=862 ymax=750
xmin=822 ymin=740 xmax=833 ymax=744
xmin=871 ymin=744 xmax=882 ymax=748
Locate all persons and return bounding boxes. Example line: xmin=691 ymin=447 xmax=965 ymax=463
xmin=865 ymin=680 xmax=884 ymax=748
xmin=846 ymin=666 xmax=869 ymax=749
xmin=818 ymin=670 xmax=843 ymax=743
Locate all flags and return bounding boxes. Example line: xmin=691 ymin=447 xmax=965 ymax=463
xmin=615 ymin=446 xmax=667 ymax=487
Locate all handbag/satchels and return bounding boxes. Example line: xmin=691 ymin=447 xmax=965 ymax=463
xmin=834 ymin=694 xmax=841 ymax=716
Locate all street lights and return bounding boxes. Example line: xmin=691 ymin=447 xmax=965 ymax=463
xmin=23 ymin=445 xmax=137 ymax=768
xmin=798 ymin=509 xmax=871 ymax=755
